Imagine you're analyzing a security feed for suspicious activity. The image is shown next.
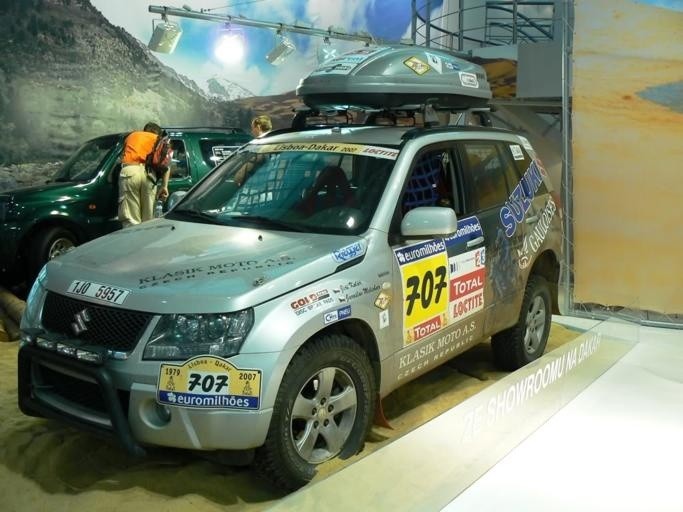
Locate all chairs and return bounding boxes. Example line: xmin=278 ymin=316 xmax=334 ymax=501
xmin=402 ymin=157 xmax=449 ymax=216
xmin=304 ymin=165 xmax=353 ymax=220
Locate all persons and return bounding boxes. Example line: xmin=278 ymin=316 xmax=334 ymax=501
xmin=234 ymin=114 xmax=280 ymax=205
xmin=118 ymin=123 xmax=171 ymax=228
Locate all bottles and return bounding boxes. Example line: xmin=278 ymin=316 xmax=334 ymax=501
xmin=155 ymin=199 xmax=162 ymax=217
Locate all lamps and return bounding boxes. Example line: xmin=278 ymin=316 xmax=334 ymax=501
xmin=148 ymin=3 xmax=372 ymax=66
